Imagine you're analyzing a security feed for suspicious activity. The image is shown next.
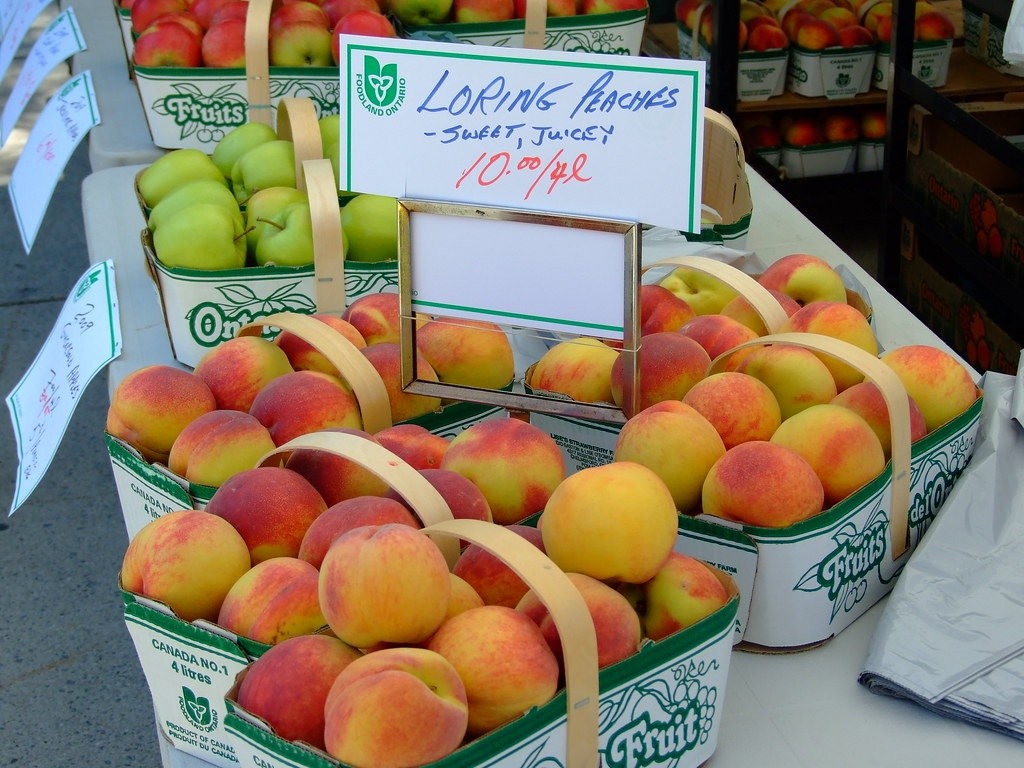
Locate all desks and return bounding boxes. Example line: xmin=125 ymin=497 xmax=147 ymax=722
xmin=69 ymin=158 xmax=998 ymax=768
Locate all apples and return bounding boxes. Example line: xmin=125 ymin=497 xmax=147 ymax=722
xmin=115 ymin=0 xmax=646 ymax=67
xmin=138 ymin=114 xmax=401 ymax=268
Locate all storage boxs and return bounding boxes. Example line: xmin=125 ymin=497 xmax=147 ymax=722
xmin=523 ymin=248 xmax=873 ymax=478
xmin=110 ymin=0 xmax=135 ymax=78
xmin=790 ymin=42 xmax=877 ymax=98
xmin=693 ymin=103 xmax=754 ymax=249
xmin=223 ymin=557 xmax=749 ymax=768
xmin=965 ymin=10 xmax=1024 ymax=79
xmin=137 ymin=215 xmax=399 ymax=371
xmin=132 ymin=30 xmax=342 ymax=152
xmin=110 ymin=507 xmax=545 ymax=768
xmin=104 ymin=326 xmax=518 ymax=546
xmin=132 ymin=160 xmax=161 ymax=216
xmin=394 ymin=0 xmax=653 ymax=56
xmin=677 ymin=21 xmax=787 ymax=103
xmin=862 ymin=143 xmax=882 ymax=173
xmin=876 ymin=37 xmax=955 ymax=91
xmin=893 ymin=217 xmax=1024 ymax=373
xmin=677 ymin=366 xmax=988 ymax=654
xmin=783 ymin=140 xmax=860 ymax=179
xmin=753 ymin=143 xmax=783 ymax=168
xmin=904 ymin=103 xmax=1024 ymax=283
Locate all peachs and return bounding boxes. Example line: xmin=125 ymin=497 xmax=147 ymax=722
xmin=106 ymin=254 xmax=976 ymax=768
xmin=676 ymin=0 xmax=953 ymax=50
xmin=735 ymin=109 xmax=887 ymax=148
